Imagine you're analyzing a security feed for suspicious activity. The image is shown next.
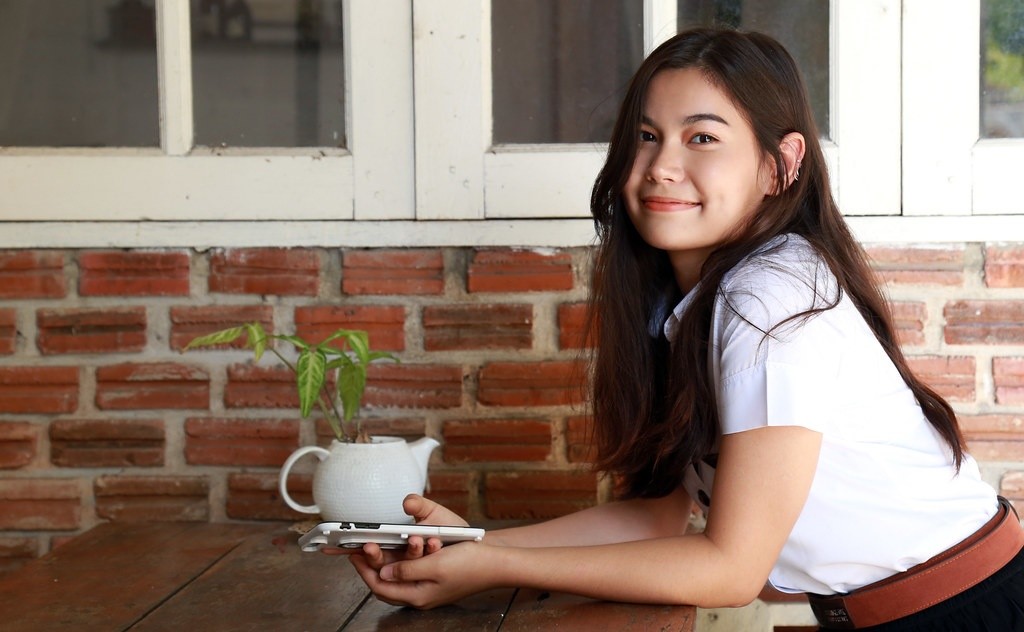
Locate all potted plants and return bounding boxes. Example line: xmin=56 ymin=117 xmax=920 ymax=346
xmin=178 ymin=321 xmax=440 ymax=524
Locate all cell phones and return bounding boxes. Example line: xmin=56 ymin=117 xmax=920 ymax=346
xmin=296 ymin=522 xmax=485 ymax=551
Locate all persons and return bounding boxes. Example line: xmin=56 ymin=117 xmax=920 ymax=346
xmin=321 ymin=29 xmax=1024 ymax=632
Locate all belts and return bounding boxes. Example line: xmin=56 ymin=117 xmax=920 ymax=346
xmin=807 ymin=495 xmax=1024 ymax=631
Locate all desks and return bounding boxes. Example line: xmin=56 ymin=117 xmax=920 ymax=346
xmin=0 ymin=520 xmax=698 ymax=632
